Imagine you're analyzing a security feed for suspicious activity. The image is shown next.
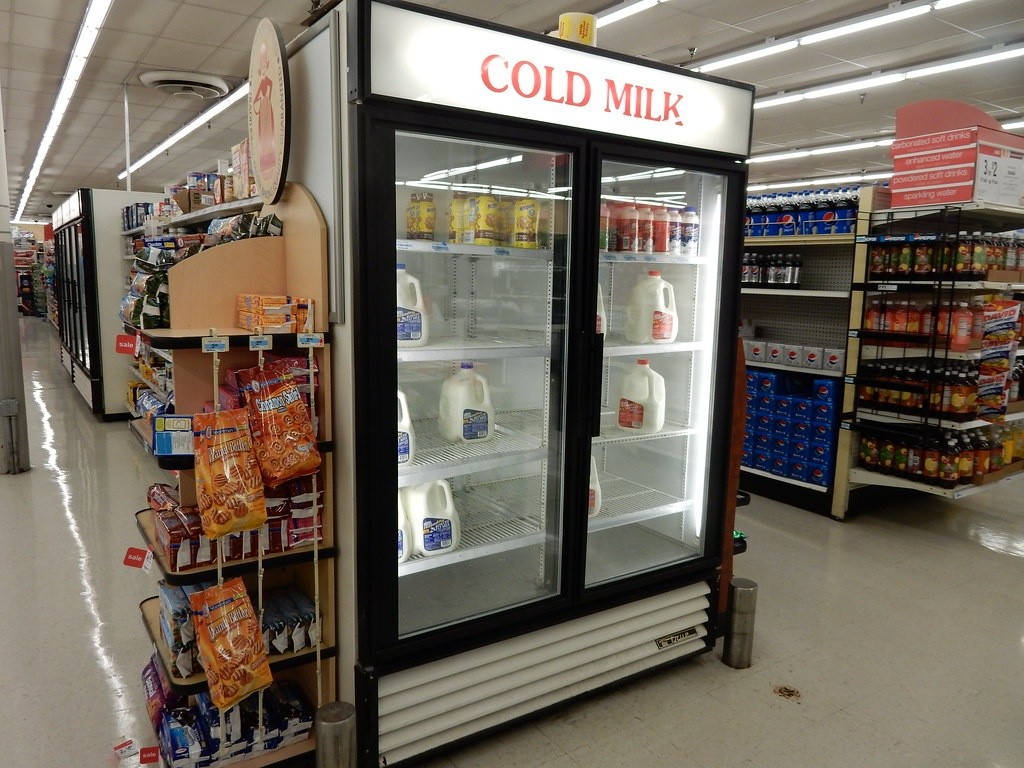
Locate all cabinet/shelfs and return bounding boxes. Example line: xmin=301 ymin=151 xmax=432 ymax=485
xmin=11 ymin=231 xmax=59 ymax=331
xmin=848 ymin=200 xmax=1024 ymax=500
xmin=738 ymin=187 xmax=891 ymax=520
xmin=127 ymin=181 xmax=339 ymax=768
xmin=120 ymin=194 xmax=265 ymax=481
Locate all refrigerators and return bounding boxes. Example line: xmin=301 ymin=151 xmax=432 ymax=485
xmin=51 ymin=188 xmax=171 ymax=423
xmin=269 ymin=1 xmax=754 ymax=768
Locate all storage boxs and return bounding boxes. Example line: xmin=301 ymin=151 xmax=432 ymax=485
xmin=122 ymin=172 xmax=225 ymax=255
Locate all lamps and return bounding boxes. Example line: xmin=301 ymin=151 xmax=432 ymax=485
xmin=117 ymin=79 xmax=249 ymax=180
xmin=546 ymin=0 xmax=1024 ymax=192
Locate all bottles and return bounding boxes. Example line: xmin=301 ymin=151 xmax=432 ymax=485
xmin=599 ymin=199 xmax=699 ymax=255
xmin=396 ymin=264 xmax=429 ymax=347
xmin=439 ymin=362 xmax=494 ymax=443
xmin=397 ymin=389 xmax=416 ymax=466
xmin=616 ymin=358 xmax=665 ymax=433
xmin=596 ymin=282 xmax=606 ymax=340
xmin=872 ymin=232 xmax=1024 ymax=280
xmin=744 ymin=182 xmax=888 ymax=235
xmin=743 ymin=253 xmax=802 ymax=289
xmin=858 ymin=359 xmax=1024 ymax=423
xmin=626 ymin=270 xmax=678 ymax=344
xmin=397 ymin=479 xmax=460 ymax=565
xmin=408 ymin=192 xmax=540 ymax=249
xmin=857 ymin=427 xmax=1012 ymax=490
xmin=866 ymin=299 xmax=983 ymax=351
xmin=588 ymin=454 xmax=601 ymax=518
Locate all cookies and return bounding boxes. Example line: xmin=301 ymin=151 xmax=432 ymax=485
xmin=204 ymin=620 xmax=264 ymax=699
xmin=198 ymin=455 xmax=262 ymax=527
